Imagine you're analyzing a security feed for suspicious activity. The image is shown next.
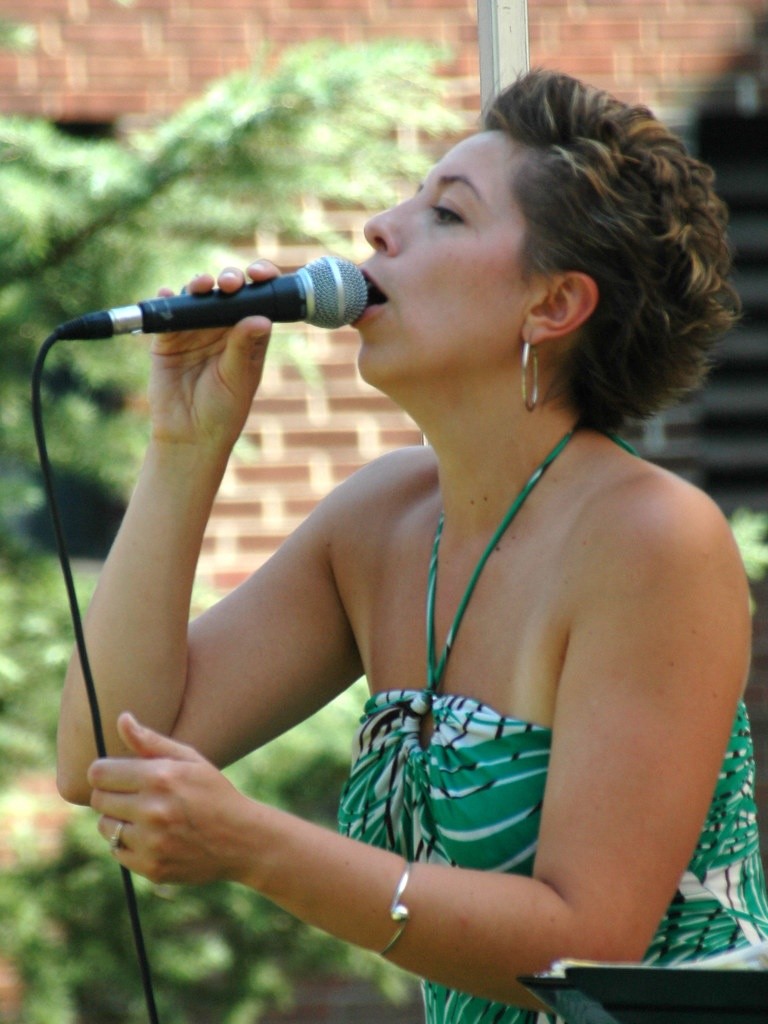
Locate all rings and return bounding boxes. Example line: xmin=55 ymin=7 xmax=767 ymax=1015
xmin=111 ymin=822 xmax=123 ymax=854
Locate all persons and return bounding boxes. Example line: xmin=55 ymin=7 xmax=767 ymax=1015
xmin=55 ymin=64 xmax=768 ymax=1024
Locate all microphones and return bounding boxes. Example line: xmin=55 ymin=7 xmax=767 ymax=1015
xmin=54 ymin=255 xmax=370 ymax=341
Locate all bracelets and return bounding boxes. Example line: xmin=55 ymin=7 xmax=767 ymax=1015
xmin=378 ymin=857 xmax=411 ymax=956
